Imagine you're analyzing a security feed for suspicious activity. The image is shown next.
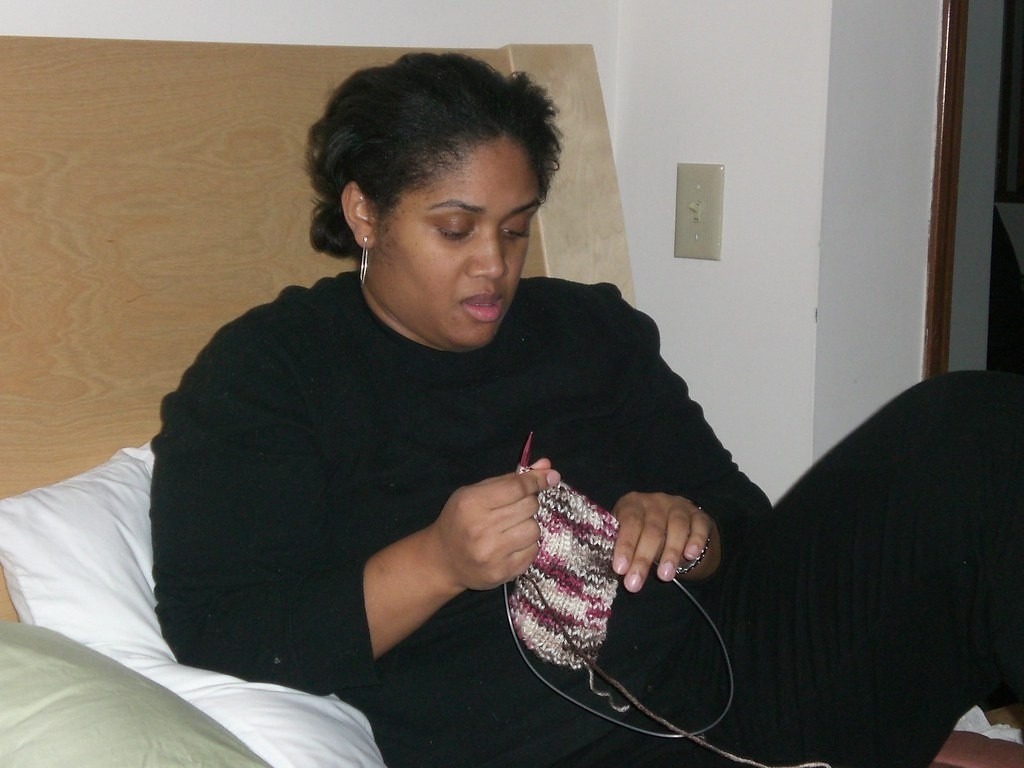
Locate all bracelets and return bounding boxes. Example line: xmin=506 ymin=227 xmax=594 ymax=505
xmin=649 ymin=501 xmax=713 ymax=574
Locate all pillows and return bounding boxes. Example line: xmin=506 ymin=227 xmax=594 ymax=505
xmin=0 ymin=442 xmax=387 ymax=768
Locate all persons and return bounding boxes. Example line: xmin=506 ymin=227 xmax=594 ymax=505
xmin=150 ymin=54 xmax=1024 ymax=768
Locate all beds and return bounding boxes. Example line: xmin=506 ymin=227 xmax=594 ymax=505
xmin=0 ymin=36 xmax=1024 ymax=768
